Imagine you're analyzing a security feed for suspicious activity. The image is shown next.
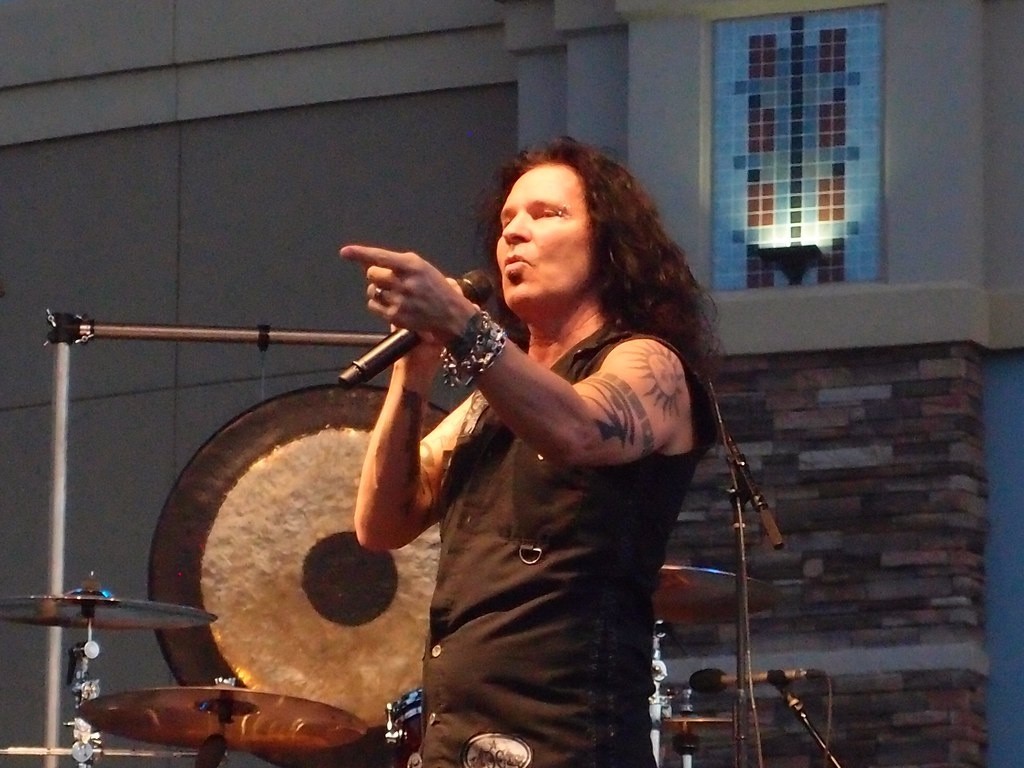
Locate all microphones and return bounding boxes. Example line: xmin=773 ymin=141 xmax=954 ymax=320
xmin=337 ymin=269 xmax=493 ymax=392
xmin=723 ymin=426 xmax=785 ymax=551
xmin=689 ymin=668 xmax=820 ymax=694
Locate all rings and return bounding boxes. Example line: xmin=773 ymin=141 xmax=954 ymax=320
xmin=375 ymin=288 xmax=384 ymax=301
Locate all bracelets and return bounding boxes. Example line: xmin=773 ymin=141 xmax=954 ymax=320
xmin=441 ymin=312 xmax=506 ymax=388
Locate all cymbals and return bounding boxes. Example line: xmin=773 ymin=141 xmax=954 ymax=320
xmin=652 ymin=564 xmax=779 ymax=627
xmin=1 ymin=589 xmax=219 ymax=632
xmin=77 ymin=684 xmax=370 ymax=755
xmin=663 ymin=717 xmax=782 ymax=737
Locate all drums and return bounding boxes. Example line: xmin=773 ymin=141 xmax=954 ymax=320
xmin=385 ymin=685 xmax=425 ymax=768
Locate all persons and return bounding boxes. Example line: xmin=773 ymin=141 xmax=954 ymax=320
xmin=341 ymin=135 xmax=724 ymax=768
